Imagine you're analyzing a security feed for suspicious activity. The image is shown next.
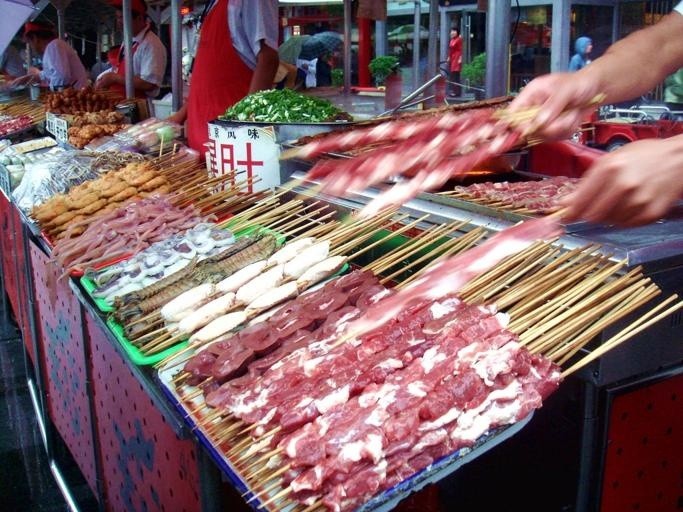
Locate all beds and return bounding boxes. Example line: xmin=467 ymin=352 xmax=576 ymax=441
xmin=386 ymin=24 xmax=428 ymax=40
xmin=278 ymin=34 xmax=315 ymax=65
xmin=296 ymin=30 xmax=343 ymax=62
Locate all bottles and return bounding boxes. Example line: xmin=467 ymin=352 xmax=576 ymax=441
xmin=116 ymin=0 xmax=145 ymax=14
xmin=23 ymin=20 xmax=53 ymax=40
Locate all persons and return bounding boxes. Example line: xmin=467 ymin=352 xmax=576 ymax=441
xmin=88 ymin=45 xmax=112 ymax=84
xmin=292 ymin=63 xmax=307 ymax=90
xmin=3 ymin=41 xmax=23 ymax=78
xmin=442 ymin=25 xmax=463 ymax=99
xmin=161 ymin=1 xmax=279 ymax=168
xmin=6 ymin=21 xmax=87 ymax=93
xmin=314 ymin=52 xmax=333 ymax=88
xmin=664 ymin=67 xmax=682 ymax=111
xmin=497 ymin=0 xmax=682 ymax=231
xmin=567 ymin=36 xmax=594 ymax=74
xmin=94 ymin=0 xmax=168 ymax=120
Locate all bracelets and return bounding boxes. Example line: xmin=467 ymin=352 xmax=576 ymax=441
xmin=216 ymin=112 xmax=379 ymax=185
xmin=152 ymin=100 xmax=174 ymax=121
